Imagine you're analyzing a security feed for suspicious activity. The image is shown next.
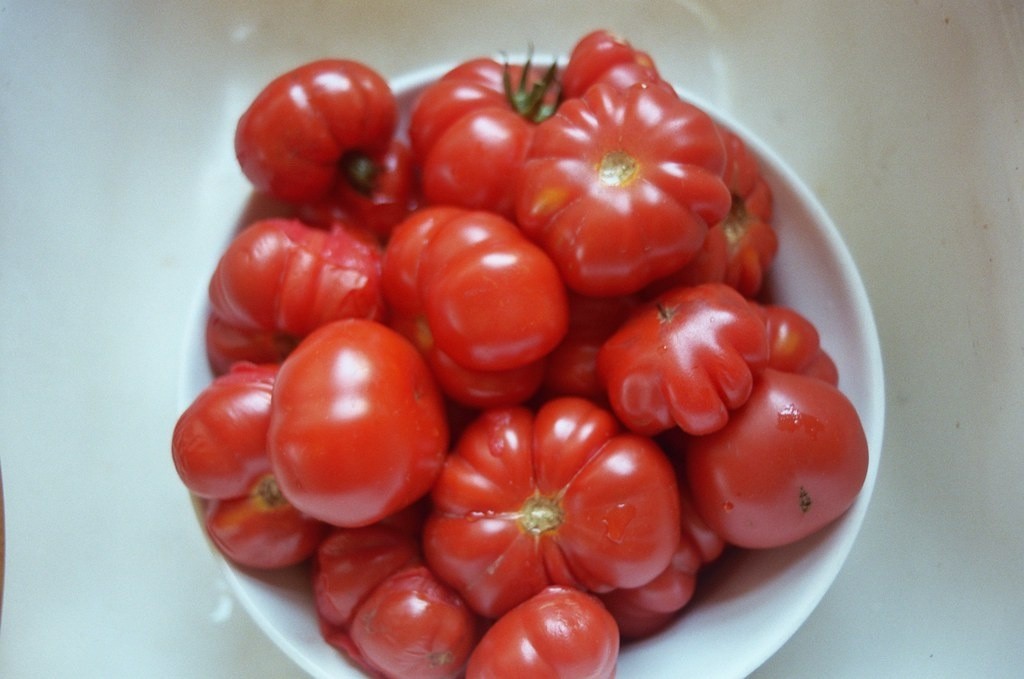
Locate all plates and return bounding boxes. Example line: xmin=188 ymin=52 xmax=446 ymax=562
xmin=169 ymin=53 xmax=886 ymax=679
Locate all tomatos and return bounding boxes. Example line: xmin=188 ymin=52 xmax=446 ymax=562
xmin=173 ymin=25 xmax=867 ymax=679
